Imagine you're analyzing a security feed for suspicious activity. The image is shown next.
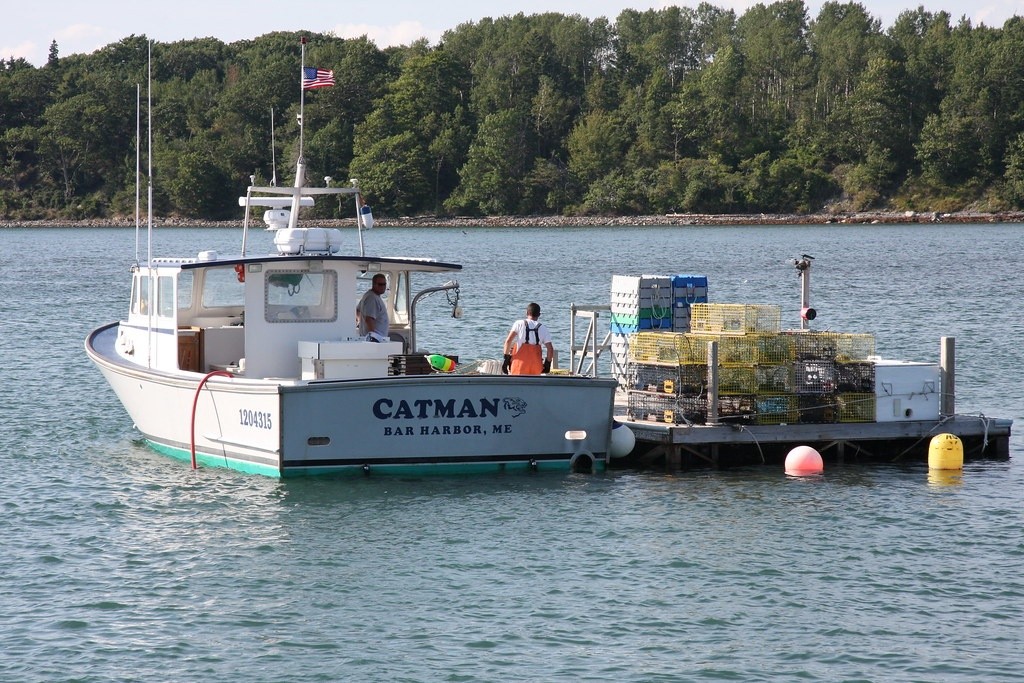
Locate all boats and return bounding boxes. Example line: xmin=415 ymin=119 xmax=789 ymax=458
xmin=82 ymin=34 xmax=619 ymax=483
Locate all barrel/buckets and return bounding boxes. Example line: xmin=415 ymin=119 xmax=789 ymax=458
xmin=239 ymin=358 xmax=245 ymax=369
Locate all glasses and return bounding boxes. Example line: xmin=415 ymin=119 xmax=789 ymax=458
xmin=376 ymin=283 xmax=386 ymax=286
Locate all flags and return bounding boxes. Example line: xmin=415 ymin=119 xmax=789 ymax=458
xmin=302 ymin=66 xmax=335 ymax=89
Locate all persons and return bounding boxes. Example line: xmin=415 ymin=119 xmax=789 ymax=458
xmin=356 ymin=273 xmax=389 ymax=337
xmin=502 ymin=303 xmax=553 ymax=375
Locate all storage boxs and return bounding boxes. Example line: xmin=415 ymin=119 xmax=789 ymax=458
xmin=385 ymin=354 xmax=458 ymax=377
xmin=275 ymin=228 xmax=343 ymax=254
xmin=611 ymin=274 xmax=708 ymax=396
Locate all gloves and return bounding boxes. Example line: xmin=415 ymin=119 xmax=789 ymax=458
xmin=541 ymin=358 xmax=552 ymax=374
xmin=502 ymin=354 xmax=512 ymax=375
